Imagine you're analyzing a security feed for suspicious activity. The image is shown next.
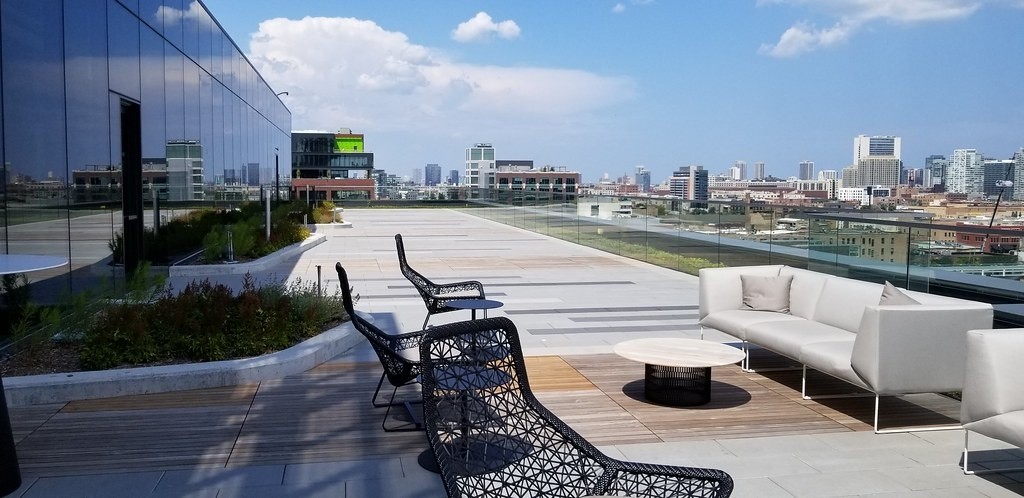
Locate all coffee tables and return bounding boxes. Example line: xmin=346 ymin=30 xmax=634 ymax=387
xmin=614 ymin=338 xmax=747 ymax=406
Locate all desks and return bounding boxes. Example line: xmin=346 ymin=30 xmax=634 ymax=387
xmin=417 ymin=300 xmax=513 ymax=475
xmin=0 ymin=253 xmax=71 ymax=497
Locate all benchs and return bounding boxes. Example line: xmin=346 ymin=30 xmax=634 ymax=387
xmin=699 ymin=265 xmax=994 ymax=434
xmin=960 ymin=328 xmax=1024 ymax=475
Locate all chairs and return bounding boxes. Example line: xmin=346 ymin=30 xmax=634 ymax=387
xmin=335 ymin=262 xmax=486 ymax=432
xmin=419 ymin=317 xmax=734 ymax=498
xmin=396 ymin=234 xmax=486 ymax=330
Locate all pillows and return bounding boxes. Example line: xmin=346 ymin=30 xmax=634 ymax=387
xmin=879 ymin=280 xmax=921 ymax=305
xmin=739 ymin=273 xmax=793 ymax=315
